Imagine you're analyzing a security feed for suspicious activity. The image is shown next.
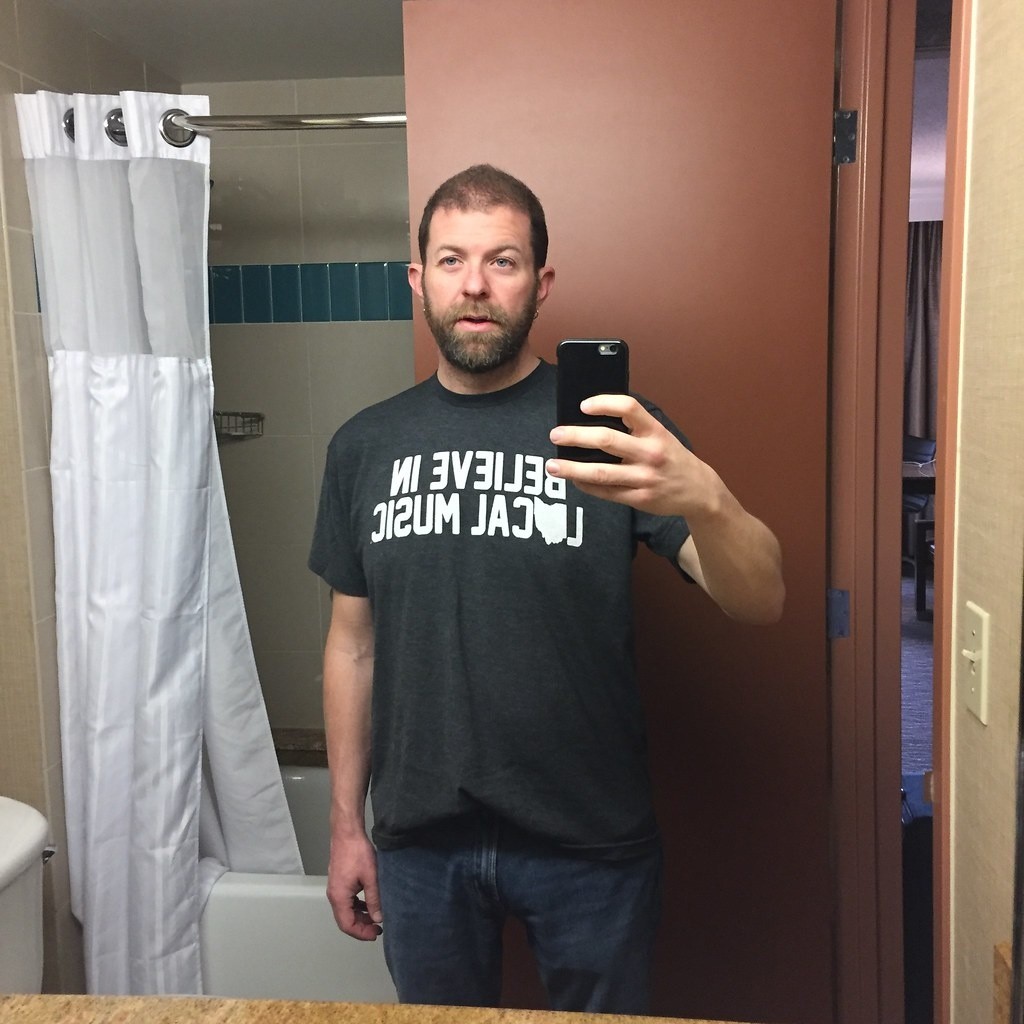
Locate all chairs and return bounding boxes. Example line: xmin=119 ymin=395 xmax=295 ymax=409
xmin=903 ymin=434 xmax=937 ymax=570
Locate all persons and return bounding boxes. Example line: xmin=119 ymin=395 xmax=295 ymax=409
xmin=310 ymin=162 xmax=786 ymax=1014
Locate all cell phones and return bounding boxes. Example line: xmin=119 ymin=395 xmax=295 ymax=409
xmin=555 ymin=338 xmax=631 ymax=466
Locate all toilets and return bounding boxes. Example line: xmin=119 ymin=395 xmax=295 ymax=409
xmin=0 ymin=794 xmax=60 ymax=995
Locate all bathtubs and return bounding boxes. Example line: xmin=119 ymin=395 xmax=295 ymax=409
xmin=193 ymin=759 xmax=401 ymax=1004
